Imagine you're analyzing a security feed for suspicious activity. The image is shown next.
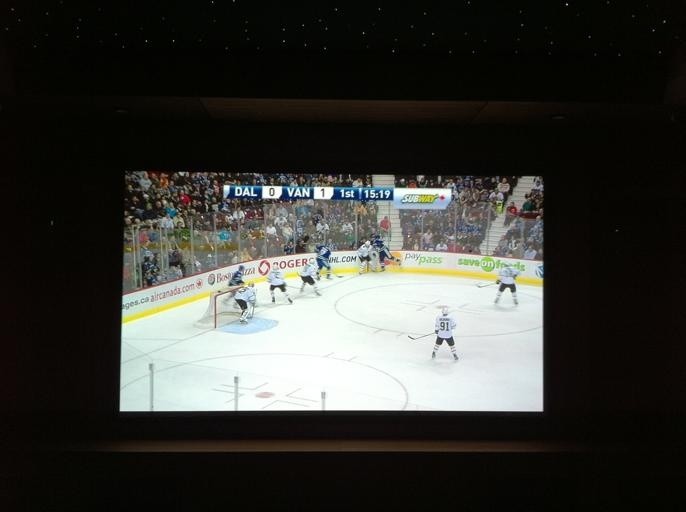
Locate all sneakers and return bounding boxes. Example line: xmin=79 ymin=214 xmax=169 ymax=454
xmin=515 ymin=302 xmax=518 ymax=305
xmin=454 ymin=354 xmax=457 ymax=359
xmin=379 ymin=268 xmax=384 ymax=271
xmin=271 ymin=298 xmax=275 ymax=302
xmin=432 ymin=351 xmax=435 ymax=358
xmin=288 ymin=299 xmax=292 ymax=302
xmin=315 ymin=291 xmax=319 ymax=295
xmin=494 ymin=298 xmax=499 ymax=303
xmin=327 ymin=275 xmax=332 ymax=279
xmin=396 ymin=258 xmax=400 ymax=262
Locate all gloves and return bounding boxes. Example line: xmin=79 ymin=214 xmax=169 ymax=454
xmin=435 ymin=330 xmax=438 ymax=334
xmin=496 ymin=280 xmax=500 ymax=284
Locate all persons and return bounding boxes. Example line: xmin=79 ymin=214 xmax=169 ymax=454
xmin=432 ymin=308 xmax=457 ymax=359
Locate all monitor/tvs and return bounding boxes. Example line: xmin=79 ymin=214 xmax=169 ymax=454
xmin=120 ymin=168 xmax=549 ymax=420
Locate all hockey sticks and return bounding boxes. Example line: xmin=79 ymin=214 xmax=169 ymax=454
xmin=475 ymin=282 xmax=497 ymax=288
xmin=330 ymin=268 xmax=344 ymax=278
xmin=407 ymin=332 xmax=435 ymax=340
xmin=247 ymin=289 xmax=257 ymax=319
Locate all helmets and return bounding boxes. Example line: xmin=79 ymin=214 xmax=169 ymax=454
xmin=327 ymin=244 xmax=331 ymax=249
xmin=238 ymin=266 xmax=244 ymax=271
xmin=503 ymin=262 xmax=509 ymax=267
xmin=309 ymin=259 xmax=314 ymax=264
xmin=248 ymin=282 xmax=254 ymax=287
xmin=442 ymin=308 xmax=448 ymax=314
xmin=364 ymin=234 xmax=379 ymax=247
xmin=273 ymin=264 xmax=277 ymax=269
